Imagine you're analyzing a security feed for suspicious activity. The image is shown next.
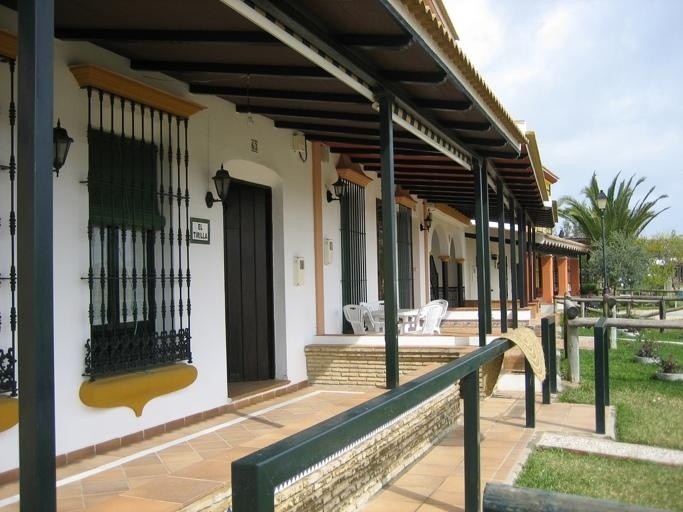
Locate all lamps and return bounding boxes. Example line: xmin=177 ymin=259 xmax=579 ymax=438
xmin=327 ymin=176 xmax=347 ymax=202
xmin=420 ymin=214 xmax=433 ymax=231
xmin=206 ymin=163 xmax=233 ymax=207
xmin=53 ymin=117 xmax=74 ymax=177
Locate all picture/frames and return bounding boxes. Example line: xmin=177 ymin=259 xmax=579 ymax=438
xmin=190 ymin=218 xmax=210 ymax=244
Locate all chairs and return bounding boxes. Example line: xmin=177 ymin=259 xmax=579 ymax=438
xmin=343 ymin=299 xmax=448 ymax=336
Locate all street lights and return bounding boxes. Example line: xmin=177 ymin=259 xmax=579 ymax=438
xmin=595 ymin=189 xmax=609 ymax=295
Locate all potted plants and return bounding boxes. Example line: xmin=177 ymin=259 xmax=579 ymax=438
xmin=657 ymin=353 xmax=683 ymax=381
xmin=633 ymin=337 xmax=660 ymax=364
